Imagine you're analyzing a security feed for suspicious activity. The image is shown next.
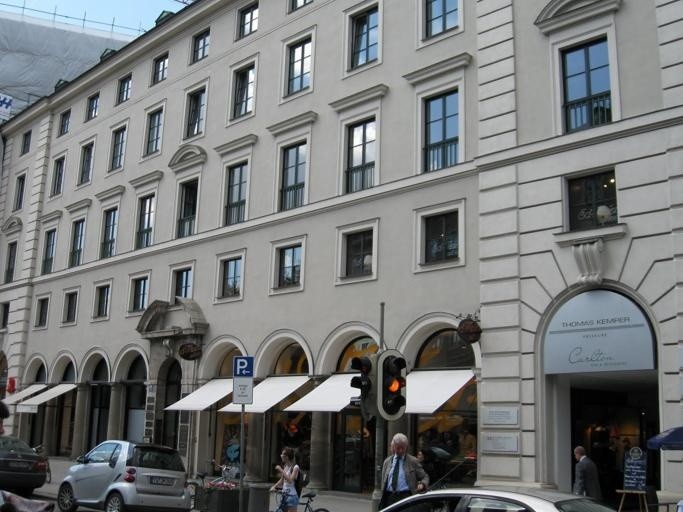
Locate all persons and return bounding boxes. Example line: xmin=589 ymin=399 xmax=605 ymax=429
xmin=376 ymin=433 xmax=429 ymax=512
xmin=574 ymin=446 xmax=601 ymax=499
xmin=269 ymin=447 xmax=303 ymax=512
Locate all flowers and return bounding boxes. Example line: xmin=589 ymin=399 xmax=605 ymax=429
xmin=204 ymin=480 xmax=238 ymax=493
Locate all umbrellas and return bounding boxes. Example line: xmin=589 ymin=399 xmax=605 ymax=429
xmin=646 ymin=426 xmax=683 ymax=450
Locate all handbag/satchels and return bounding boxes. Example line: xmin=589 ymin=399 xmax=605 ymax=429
xmin=296 ymin=471 xmax=310 ymax=488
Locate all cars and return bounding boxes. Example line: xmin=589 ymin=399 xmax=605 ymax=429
xmin=56 ymin=437 xmax=192 ymax=512
xmin=0 ymin=434 xmax=48 ymax=496
xmin=375 ymin=483 xmax=615 ymax=512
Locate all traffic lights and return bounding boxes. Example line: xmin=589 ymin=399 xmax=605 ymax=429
xmin=349 ymin=344 xmax=408 ymax=424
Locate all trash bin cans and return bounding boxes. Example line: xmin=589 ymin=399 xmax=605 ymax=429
xmin=194 ymin=486 xmax=249 ymax=512
xmin=248 ymin=483 xmax=270 ymax=512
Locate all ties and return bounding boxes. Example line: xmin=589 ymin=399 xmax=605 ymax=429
xmin=392 ymin=458 xmax=399 ymax=491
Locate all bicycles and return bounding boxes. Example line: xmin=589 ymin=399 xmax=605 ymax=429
xmin=188 ymin=458 xmax=240 ymax=510
xmin=268 ymin=484 xmax=330 ymax=511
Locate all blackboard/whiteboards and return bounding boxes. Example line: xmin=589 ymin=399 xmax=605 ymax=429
xmin=624 ymin=448 xmax=646 ymax=487
